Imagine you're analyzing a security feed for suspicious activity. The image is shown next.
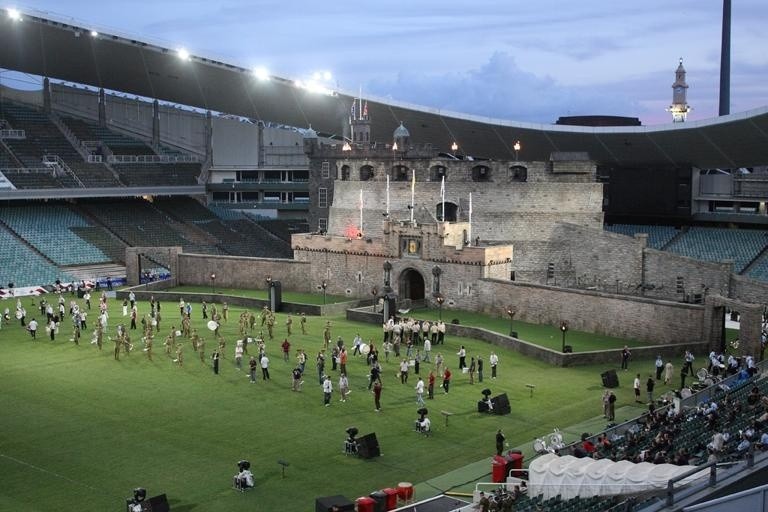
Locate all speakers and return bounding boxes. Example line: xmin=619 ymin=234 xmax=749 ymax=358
xmin=491 ymin=392 xmax=511 ymax=415
xmin=316 ymin=495 xmax=355 ymax=512
xmin=355 ymin=432 xmax=380 ymax=458
xmin=149 ymin=494 xmax=169 ymax=512
xmin=600 ymin=369 xmax=619 ymax=387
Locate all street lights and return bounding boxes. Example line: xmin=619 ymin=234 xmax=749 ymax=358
xmin=323 ymin=284 xmax=327 ymax=304
xmin=266 ymin=275 xmax=272 ymax=301
xmin=560 ymin=322 xmax=568 ymax=353
xmin=145 ymin=272 xmax=148 ymax=292
xmin=343 ymin=143 xmax=352 ymax=160
xmin=393 ymin=142 xmax=397 ymax=161
xmin=452 ymin=143 xmax=458 ymax=160
xmin=371 ymin=287 xmax=378 ymax=313
xmin=210 ymin=272 xmax=216 ymax=293
xmin=514 ymin=141 xmax=520 ymax=160
xmin=436 ymin=294 xmax=445 ymax=320
xmin=508 ymin=309 xmax=515 ymax=336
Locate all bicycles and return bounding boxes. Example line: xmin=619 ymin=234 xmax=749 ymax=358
xmin=724 ymin=344 xmax=734 ymax=355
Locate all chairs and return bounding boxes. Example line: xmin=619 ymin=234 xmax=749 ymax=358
xmin=470 ymin=374 xmax=768 ymax=512
xmin=398 ymin=299 xmax=416 ymax=316
xmin=603 ymin=223 xmax=768 ymax=280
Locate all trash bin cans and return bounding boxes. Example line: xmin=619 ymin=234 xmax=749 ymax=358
xmin=78 ymin=286 xmax=86 ymax=299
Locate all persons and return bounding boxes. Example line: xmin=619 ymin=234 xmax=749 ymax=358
xmin=332 ymin=504 xmax=339 ymax=512
xmin=496 ymin=429 xmax=506 ymax=457
xmin=569 ymin=311 xmax=768 ymax=466
xmin=479 ymin=481 xmax=528 ymax=512
xmin=352 ymin=314 xmax=498 ymax=410
xmin=2 ymin=277 xmax=162 ymax=359
xmin=165 ymin=296 xmax=274 ymax=383
xmin=280 ymin=312 xmax=349 ymax=407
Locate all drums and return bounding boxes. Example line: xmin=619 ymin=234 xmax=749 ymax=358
xmin=359 ymin=343 xmax=370 ymax=355
xmin=532 ymin=439 xmax=546 ymax=453
xmin=550 ymin=435 xmax=562 ymax=446
xmin=696 ymin=368 xmax=707 ymax=379
xmin=207 ymin=320 xmax=218 ymax=330
xmin=398 ymin=482 xmax=412 ymax=500
